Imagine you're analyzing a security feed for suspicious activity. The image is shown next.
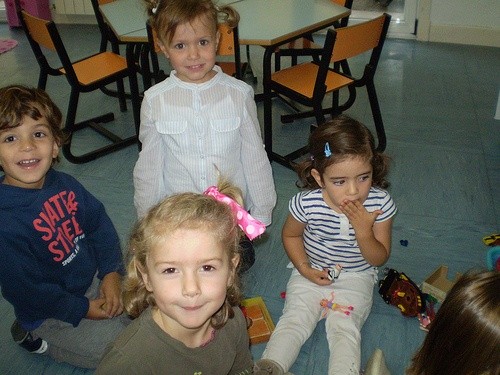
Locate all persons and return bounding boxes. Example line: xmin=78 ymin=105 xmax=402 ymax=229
xmin=91 ymin=182 xmax=264 ymax=375
xmin=133 ymin=0 xmax=276 ymax=272
xmin=409 ymin=270 xmax=500 ymax=375
xmin=0 ymin=85 xmax=131 ymax=369
xmin=255 ymin=117 xmax=398 ymax=375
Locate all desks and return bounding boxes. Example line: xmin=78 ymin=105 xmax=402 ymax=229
xmin=100 ymin=0 xmax=352 ymax=162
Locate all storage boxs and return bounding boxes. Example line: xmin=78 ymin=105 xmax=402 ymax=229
xmin=422 ymin=266 xmax=463 ymax=312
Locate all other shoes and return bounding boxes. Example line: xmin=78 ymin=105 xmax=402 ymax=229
xmin=252 ymin=358 xmax=285 ymax=375
xmin=13 ymin=331 xmax=48 ymax=354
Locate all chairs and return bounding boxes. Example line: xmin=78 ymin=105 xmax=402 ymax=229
xmin=18 ymin=9 xmax=137 ymax=165
xmin=264 ymin=12 xmax=392 ymax=170
xmin=255 ymin=1 xmax=355 ymax=122
xmin=90 ymin=0 xmax=153 ymax=98
xmin=147 ymin=15 xmax=256 ymax=87
